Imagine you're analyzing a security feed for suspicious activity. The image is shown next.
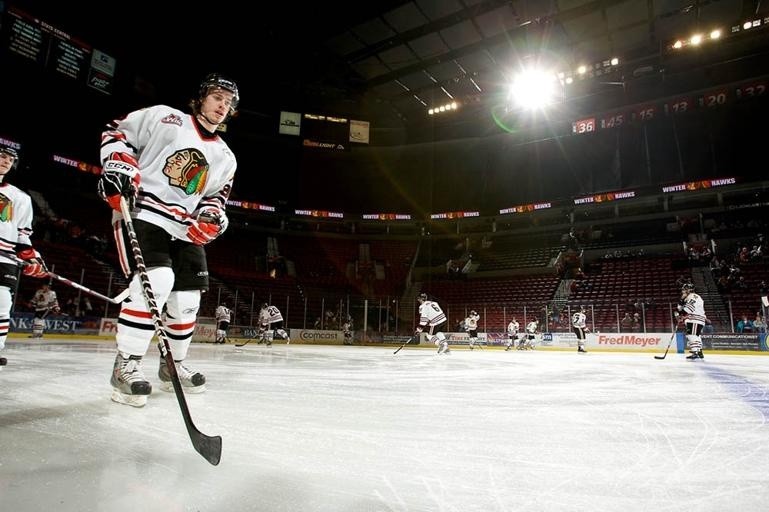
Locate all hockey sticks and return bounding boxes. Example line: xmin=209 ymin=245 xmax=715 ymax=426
xmin=119 ymin=196 xmax=222 ymax=466
xmin=587 ymin=325 xmax=601 ymax=337
xmin=655 ymin=322 xmax=679 ymax=359
xmin=235 ymin=334 xmax=259 ymax=346
xmin=0 ymin=249 xmax=129 ymax=304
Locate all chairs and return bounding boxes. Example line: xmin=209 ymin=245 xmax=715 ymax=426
xmin=444 ymin=238 xmax=769 ymax=332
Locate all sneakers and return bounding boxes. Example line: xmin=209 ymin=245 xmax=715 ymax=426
xmin=1 ymin=355 xmax=7 ymax=365
xmin=258 ymin=337 xmax=273 ymax=345
xmin=110 ymin=353 xmax=152 ymax=395
xmin=578 ymin=346 xmax=587 ymax=352
xmin=686 ymin=349 xmax=704 ymax=359
xmin=438 ymin=342 xmax=450 ymax=355
xmin=158 ymin=344 xmax=205 ymax=386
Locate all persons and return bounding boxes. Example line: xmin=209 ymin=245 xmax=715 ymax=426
xmin=257 ymin=307 xmax=269 ymax=344
xmin=415 ymin=292 xmax=451 ymax=356
xmin=63 ymin=285 xmax=122 ymax=315
xmin=621 ymin=298 xmax=656 ymax=322
xmin=28 ymin=281 xmax=62 ymax=338
xmin=571 ymin=307 xmax=589 ymax=352
xmin=507 ymin=315 xmax=519 ymax=350
xmin=214 ymin=302 xmax=235 ymax=344
xmin=753 ymin=316 xmax=768 ymax=332
xmin=736 ymin=315 xmax=753 ymax=333
xmin=314 ymin=299 xmax=354 ymax=344
xmin=96 ymin=74 xmax=242 ymax=408
xmin=259 ymin=302 xmax=290 ymax=346
xmin=675 ymin=283 xmax=707 ymax=359
xmin=464 ymin=310 xmax=480 ymax=349
xmin=604 ymin=247 xmax=646 ymax=259
xmin=0 ymin=139 xmax=50 ymax=366
xmin=688 ymin=219 xmax=768 ymax=296
xmin=523 ymin=317 xmax=538 ymax=349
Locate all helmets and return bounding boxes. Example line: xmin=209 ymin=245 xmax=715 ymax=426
xmin=192 ymin=74 xmax=239 ymax=118
xmin=417 ymin=294 xmax=427 ymax=301
xmin=0 ymin=137 xmax=21 ymax=161
xmin=682 ymin=284 xmax=695 ymax=293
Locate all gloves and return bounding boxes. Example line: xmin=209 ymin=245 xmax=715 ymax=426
xmin=16 ymin=249 xmax=48 ymax=278
xmin=98 ymin=152 xmax=141 ymax=213
xmin=187 ymin=208 xmax=229 ymax=245
